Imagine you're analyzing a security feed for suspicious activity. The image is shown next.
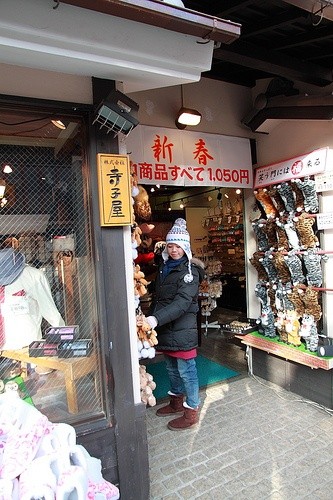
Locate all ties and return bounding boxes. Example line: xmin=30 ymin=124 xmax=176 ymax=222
xmin=0 ymin=280 xmax=5 ymax=355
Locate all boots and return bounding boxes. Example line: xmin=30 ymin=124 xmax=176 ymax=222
xmin=155 ymin=391 xmax=184 ymax=417
xmin=167 ymin=401 xmax=199 ymax=431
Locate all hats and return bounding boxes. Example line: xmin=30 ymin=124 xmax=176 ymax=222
xmin=162 ymin=217 xmax=194 ymax=284
xmin=0 ymin=247 xmax=26 ymax=286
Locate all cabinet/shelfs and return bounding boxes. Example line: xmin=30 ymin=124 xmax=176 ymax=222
xmin=198 ymin=272 xmax=220 ymax=335
xmin=207 ymin=228 xmax=243 ymax=254
xmin=241 ymin=212 xmax=333 ymax=370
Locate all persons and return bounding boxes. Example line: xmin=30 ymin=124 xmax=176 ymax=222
xmin=144 ymin=219 xmax=205 ymax=429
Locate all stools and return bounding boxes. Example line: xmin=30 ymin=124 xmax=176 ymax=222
xmin=1 ymin=347 xmax=101 ymax=416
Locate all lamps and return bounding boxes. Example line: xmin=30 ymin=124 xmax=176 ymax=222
xmin=175 ymin=84 xmax=202 ymax=130
xmin=217 ymin=189 xmax=222 ymax=200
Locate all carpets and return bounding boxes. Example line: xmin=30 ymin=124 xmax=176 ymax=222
xmin=146 ymin=353 xmax=246 ymax=409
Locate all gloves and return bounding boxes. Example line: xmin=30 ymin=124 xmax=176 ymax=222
xmin=146 ymin=315 xmax=158 ymax=330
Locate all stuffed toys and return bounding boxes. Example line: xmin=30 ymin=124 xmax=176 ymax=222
xmin=132 ymin=182 xmax=159 ymax=407
xmin=198 ymin=258 xmax=223 ymax=316
xmin=249 ymin=174 xmax=325 ymax=352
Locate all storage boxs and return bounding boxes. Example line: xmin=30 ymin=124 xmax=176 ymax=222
xmin=57 ymin=339 xmax=93 ymax=358
xmin=45 ymin=325 xmax=79 ymax=342
xmin=28 ymin=341 xmax=64 ymax=357
